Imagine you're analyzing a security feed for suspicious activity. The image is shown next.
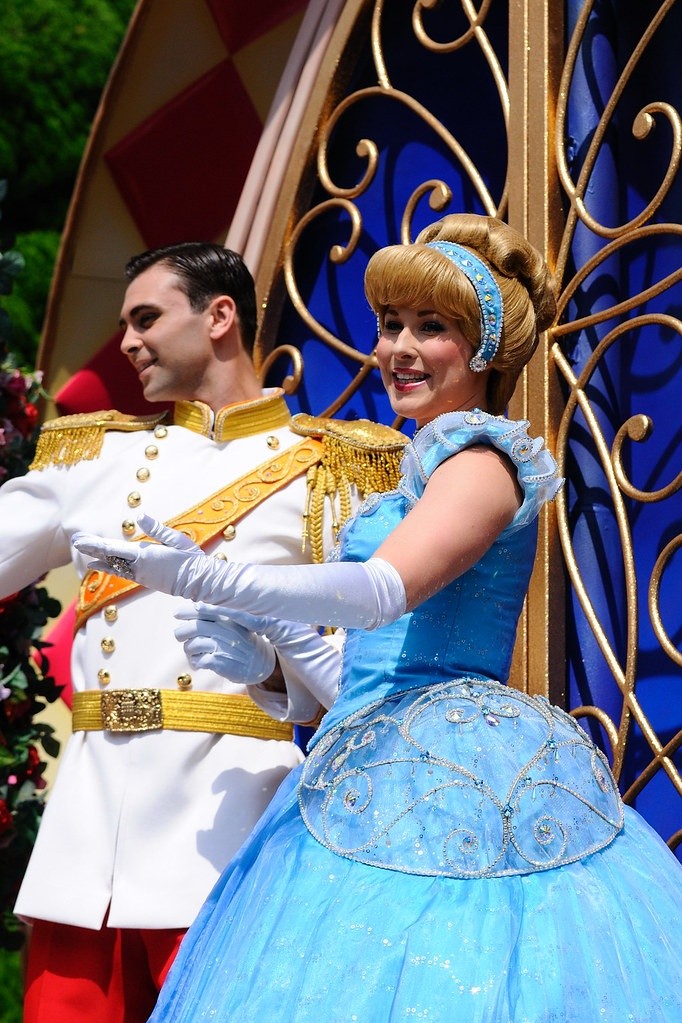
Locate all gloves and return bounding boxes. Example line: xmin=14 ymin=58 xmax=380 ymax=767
xmin=69 ymin=514 xmax=407 ymax=631
xmin=194 ymin=599 xmax=344 ymax=711
xmin=174 ymin=609 xmax=277 ymax=686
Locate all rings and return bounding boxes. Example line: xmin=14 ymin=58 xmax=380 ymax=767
xmin=104 ymin=555 xmax=134 ymax=581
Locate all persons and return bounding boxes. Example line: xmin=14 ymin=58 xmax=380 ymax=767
xmin=0 ymin=237 xmax=410 ymax=1023
xmin=66 ymin=213 xmax=681 ymax=1023
